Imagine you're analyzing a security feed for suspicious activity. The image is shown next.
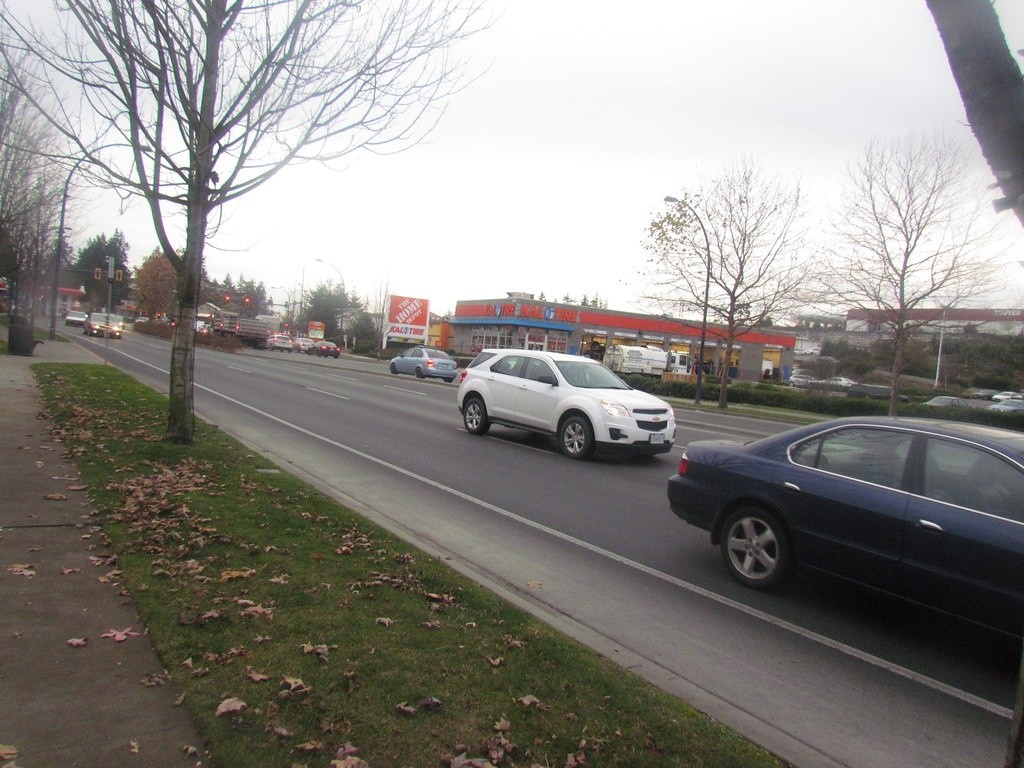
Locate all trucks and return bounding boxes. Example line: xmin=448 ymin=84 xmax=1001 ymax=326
xmin=602 ymin=344 xmax=696 ymax=380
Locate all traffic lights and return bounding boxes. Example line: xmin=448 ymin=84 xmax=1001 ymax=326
xmin=244 ymin=298 xmax=250 ymax=308
xmin=225 ymin=295 xmax=231 ymax=304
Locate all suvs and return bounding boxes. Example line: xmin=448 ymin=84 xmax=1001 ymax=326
xmin=846 ymin=382 xmax=911 ymax=406
xmin=457 ymin=348 xmax=677 ymax=462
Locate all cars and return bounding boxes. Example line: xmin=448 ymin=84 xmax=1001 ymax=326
xmin=787 ymin=366 xmax=859 ymax=389
xmin=987 ymin=399 xmax=1024 ymax=418
xmin=267 ymin=334 xmax=293 ymax=352
xmin=972 ymin=388 xmax=1001 ymax=401
xmin=290 ymin=338 xmax=316 ymax=354
xmin=667 ymin=417 xmax=1024 ymax=658
xmin=82 ymin=312 xmax=127 ymax=339
xmin=308 ymin=340 xmax=341 ymax=359
xmin=134 ymin=316 xmax=150 ymax=325
xmin=390 ymin=347 xmax=458 ymax=383
xmin=159 ymin=312 xmax=213 ymax=336
xmin=923 ymin=395 xmax=972 ymax=411
xmin=65 ymin=311 xmax=89 ymax=327
xmin=992 ymin=391 xmax=1024 ymax=401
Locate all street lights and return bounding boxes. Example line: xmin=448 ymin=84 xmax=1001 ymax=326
xmin=663 ymin=195 xmax=711 ymax=404
xmin=48 ymin=144 xmax=153 ymax=341
xmin=316 ymin=258 xmax=345 ymax=335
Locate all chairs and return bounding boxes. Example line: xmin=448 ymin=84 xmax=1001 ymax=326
xmin=925 ymin=453 xmax=956 ymax=505
